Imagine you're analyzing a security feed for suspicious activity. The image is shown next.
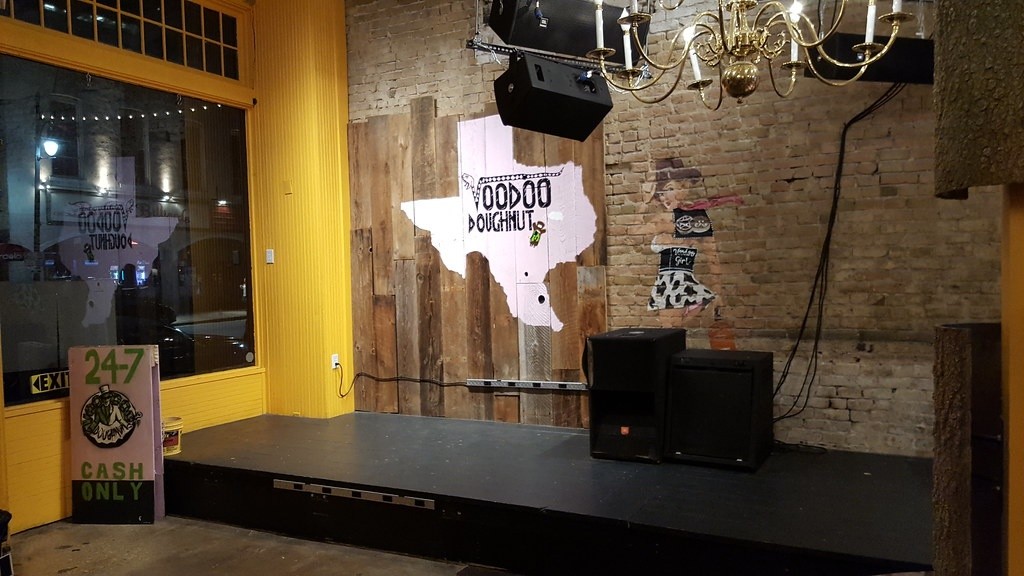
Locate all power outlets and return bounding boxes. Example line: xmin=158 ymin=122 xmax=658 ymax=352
xmin=331 ymin=353 xmax=339 ymax=371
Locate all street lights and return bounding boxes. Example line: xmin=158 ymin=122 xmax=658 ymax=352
xmin=33 ymin=122 xmax=59 ymax=277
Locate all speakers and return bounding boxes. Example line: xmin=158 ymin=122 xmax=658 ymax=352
xmin=584 ymin=328 xmax=776 ymax=473
xmin=488 ymin=0 xmax=652 ymax=142
xmin=803 ymin=32 xmax=935 ymax=86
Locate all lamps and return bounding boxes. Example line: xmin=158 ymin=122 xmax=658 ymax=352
xmin=803 ymin=32 xmax=934 ymax=84
xmin=492 ymin=47 xmax=614 ymax=142
xmin=585 ymin=0 xmax=920 ymax=112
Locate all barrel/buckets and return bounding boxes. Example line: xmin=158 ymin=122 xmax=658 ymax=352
xmin=162 ymin=417 xmax=183 ymax=457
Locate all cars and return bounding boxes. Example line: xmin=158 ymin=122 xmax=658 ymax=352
xmin=58 ymin=319 xmax=254 ymax=383
xmin=79 ymin=288 xmax=175 ymax=326
